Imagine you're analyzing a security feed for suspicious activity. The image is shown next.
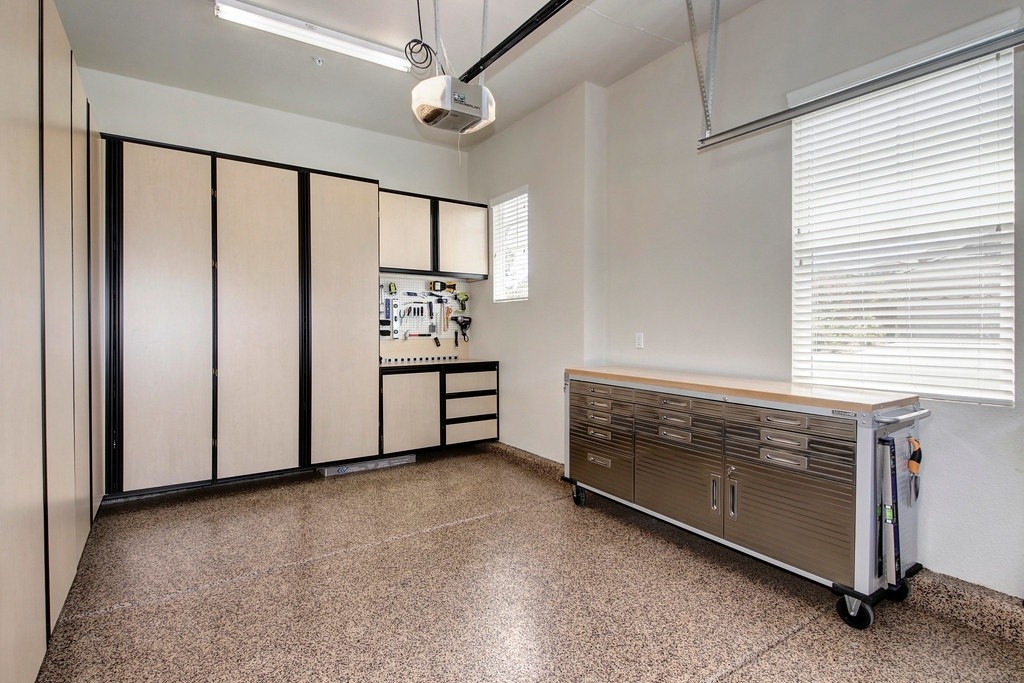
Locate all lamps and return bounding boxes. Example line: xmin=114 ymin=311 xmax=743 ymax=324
xmin=214 ymin=0 xmax=411 ymax=72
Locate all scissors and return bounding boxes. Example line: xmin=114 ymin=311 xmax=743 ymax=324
xmin=446 ymin=307 xmax=452 ymax=328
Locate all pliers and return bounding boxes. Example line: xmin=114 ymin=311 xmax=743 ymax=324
xmin=399 ymin=310 xmax=405 ymax=325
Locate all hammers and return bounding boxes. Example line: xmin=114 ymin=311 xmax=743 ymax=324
xmin=437 ymin=299 xmax=448 ymax=331
xmin=404 ymin=330 xmax=431 ymax=341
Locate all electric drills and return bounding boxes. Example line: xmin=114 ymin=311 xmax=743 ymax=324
xmin=448 ymin=316 xmax=472 ymax=342
xmin=447 ymin=292 xmax=469 ymax=310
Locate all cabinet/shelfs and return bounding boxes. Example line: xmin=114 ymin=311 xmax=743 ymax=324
xmin=0 ymin=1 xmax=113 ymax=681
xmin=113 ymin=134 xmax=304 ymax=496
xmin=305 ymin=168 xmax=380 ymax=466
xmin=379 ymin=187 xmax=489 ymax=282
xmin=381 ymin=366 xmax=501 ymax=458
xmin=562 ymin=368 xmax=931 ymax=632
